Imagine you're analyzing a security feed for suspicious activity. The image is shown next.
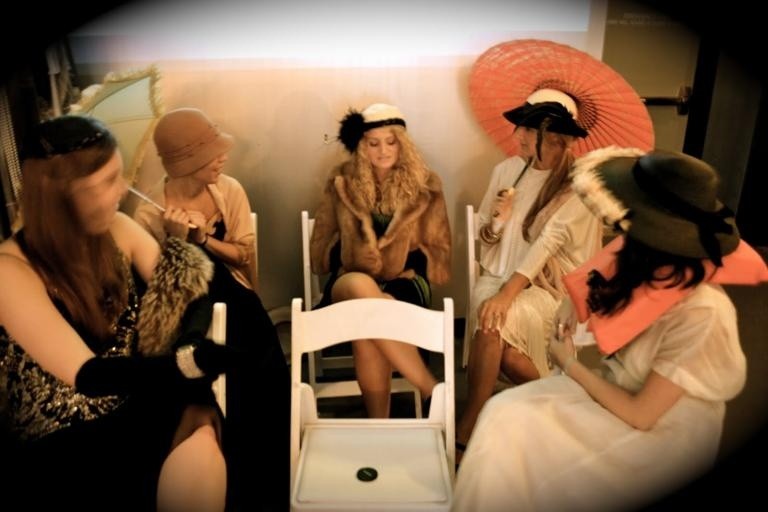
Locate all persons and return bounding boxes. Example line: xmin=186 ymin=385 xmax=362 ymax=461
xmin=308 ymin=100 xmax=453 ymax=417
xmin=133 ymin=106 xmax=258 ymax=292
xmin=0 ymin=112 xmax=231 ymax=512
xmin=455 ymin=102 xmax=606 ymax=473
xmin=451 ymin=143 xmax=751 ymax=512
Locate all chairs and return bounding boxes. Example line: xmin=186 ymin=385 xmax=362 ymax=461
xmin=288 ymin=297 xmax=455 ymax=512
xmin=462 ymin=205 xmax=597 ymax=370
xmin=300 ymin=210 xmax=422 ymax=418
xmin=204 ymin=301 xmax=227 ymax=420
xmin=249 ymin=213 xmax=258 ymax=286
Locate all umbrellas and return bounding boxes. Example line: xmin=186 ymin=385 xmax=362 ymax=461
xmin=10 ymin=64 xmax=198 ymax=236
xmin=468 ymin=38 xmax=656 ymax=197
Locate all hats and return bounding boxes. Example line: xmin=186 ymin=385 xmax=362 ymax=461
xmin=361 ymin=104 xmax=405 ymax=131
xmin=568 ymin=145 xmax=739 ymax=258
xmin=503 ymin=88 xmax=588 ymax=139
xmin=153 ymin=108 xmax=233 ymax=178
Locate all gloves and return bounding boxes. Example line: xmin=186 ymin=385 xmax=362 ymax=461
xmin=75 ymin=341 xmax=230 ymax=396
